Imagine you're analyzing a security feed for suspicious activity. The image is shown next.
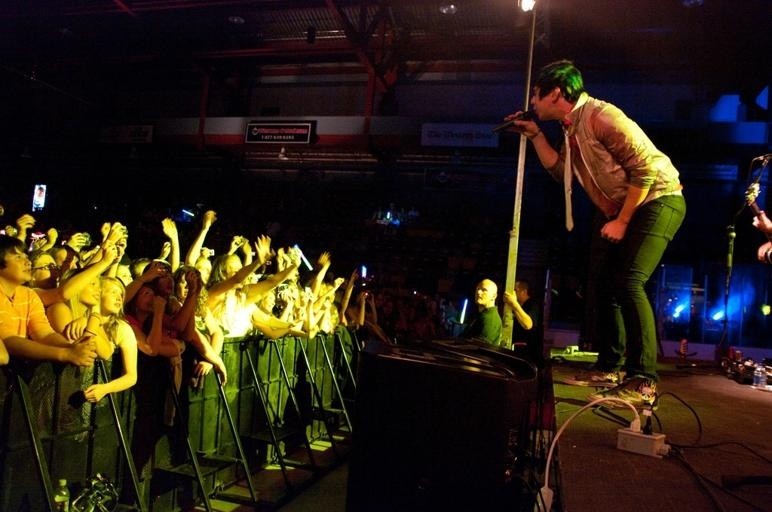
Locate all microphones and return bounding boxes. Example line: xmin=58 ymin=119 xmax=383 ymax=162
xmin=758 ymin=154 xmax=772 ymax=161
xmin=492 ymin=109 xmax=534 ymax=133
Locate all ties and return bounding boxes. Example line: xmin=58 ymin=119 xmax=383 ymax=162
xmin=564 ymin=127 xmax=574 ymax=232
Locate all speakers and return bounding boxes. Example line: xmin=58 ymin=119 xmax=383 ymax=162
xmin=655 ymin=265 xmax=746 ymax=346
xmin=343 ymin=333 xmax=540 ymax=512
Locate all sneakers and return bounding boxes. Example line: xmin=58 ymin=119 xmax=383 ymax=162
xmin=586 ymin=378 xmax=659 ymax=411
xmin=561 ymin=368 xmax=624 ymax=388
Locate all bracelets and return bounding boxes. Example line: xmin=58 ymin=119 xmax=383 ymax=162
xmin=528 ymin=127 xmax=542 ymax=142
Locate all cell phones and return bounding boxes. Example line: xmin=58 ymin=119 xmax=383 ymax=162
xmin=31 ymin=183 xmax=49 ymax=215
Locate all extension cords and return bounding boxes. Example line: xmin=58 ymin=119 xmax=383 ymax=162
xmin=616 ymin=428 xmax=671 ymax=459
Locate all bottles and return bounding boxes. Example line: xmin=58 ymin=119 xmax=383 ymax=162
xmin=753 ymin=360 xmax=767 ymax=388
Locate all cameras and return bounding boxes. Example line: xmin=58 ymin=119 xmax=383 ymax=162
xmin=183 ymin=265 xmax=195 ymax=275
xmin=157 ymin=265 xmax=169 ymax=274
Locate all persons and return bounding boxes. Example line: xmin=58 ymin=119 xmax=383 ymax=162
xmin=502 ymin=279 xmax=536 ymax=344
xmin=1 ymin=199 xmax=458 ymax=485
xmin=504 ymin=58 xmax=690 ymax=412
xmin=464 ymin=274 xmax=503 ymax=345
xmin=745 ymin=208 xmax=772 ymax=270
